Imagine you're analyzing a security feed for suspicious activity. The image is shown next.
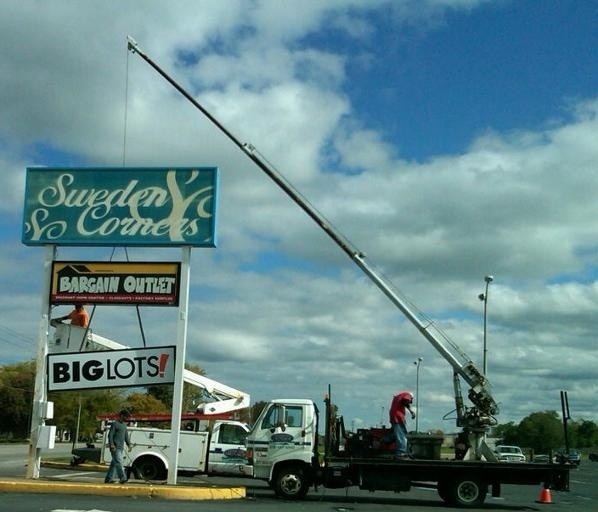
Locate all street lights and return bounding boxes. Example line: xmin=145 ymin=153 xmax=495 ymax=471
xmin=413 ymin=357 xmax=424 ymax=434
xmin=478 ymin=275 xmax=494 ymax=377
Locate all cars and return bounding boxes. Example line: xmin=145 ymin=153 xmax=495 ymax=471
xmin=493 ymin=444 xmax=526 ymax=464
xmin=556 ymin=447 xmax=581 ymax=466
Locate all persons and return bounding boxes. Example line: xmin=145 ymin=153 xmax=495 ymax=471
xmin=55 ymin=305 xmax=89 ymax=328
xmin=105 ymin=410 xmax=132 ymax=483
xmin=390 ymin=391 xmax=415 ymax=456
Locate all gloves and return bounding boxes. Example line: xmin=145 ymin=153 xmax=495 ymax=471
xmin=110 ymin=444 xmax=115 ymax=452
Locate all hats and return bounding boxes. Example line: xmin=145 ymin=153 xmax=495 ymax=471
xmin=119 ymin=410 xmax=130 ymax=417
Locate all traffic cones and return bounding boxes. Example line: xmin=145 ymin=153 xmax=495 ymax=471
xmin=536 ymin=488 xmax=556 ymax=504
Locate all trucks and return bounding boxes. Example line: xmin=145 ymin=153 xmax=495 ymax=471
xmin=242 ymin=389 xmax=577 ymax=508
xmin=100 ymin=415 xmax=251 ymax=481
